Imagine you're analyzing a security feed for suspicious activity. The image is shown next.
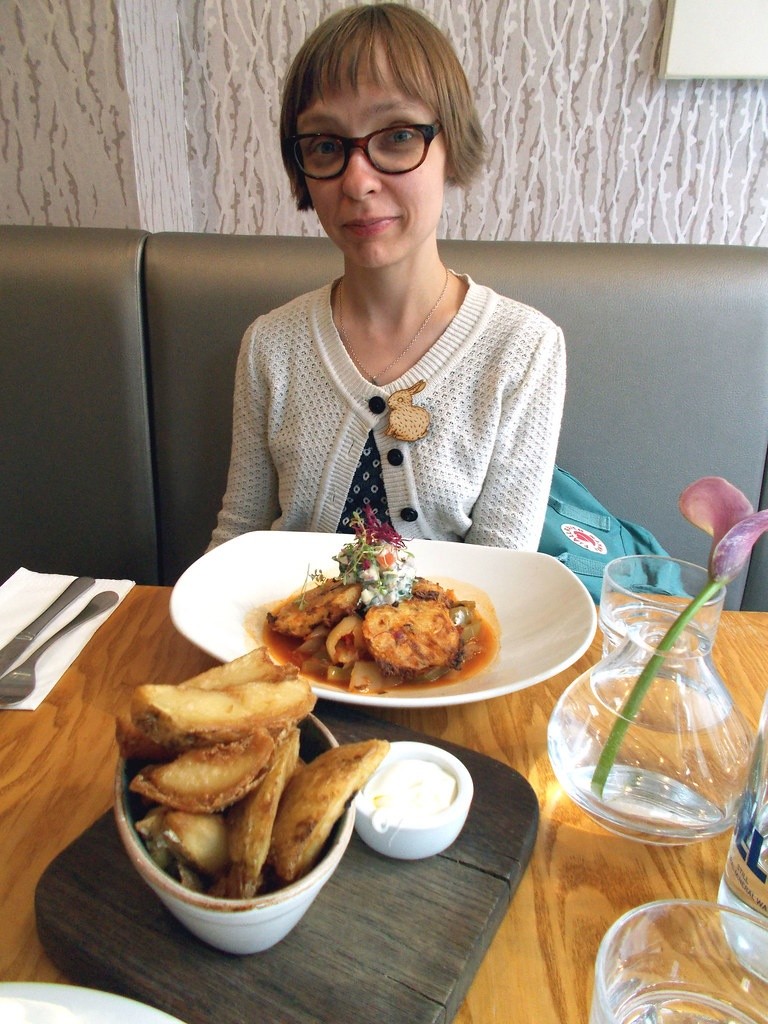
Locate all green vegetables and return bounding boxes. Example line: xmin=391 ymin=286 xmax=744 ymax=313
xmin=294 ymin=503 xmax=416 ymax=611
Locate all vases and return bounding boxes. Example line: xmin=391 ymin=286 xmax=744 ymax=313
xmin=543 ymin=554 xmax=751 ymax=846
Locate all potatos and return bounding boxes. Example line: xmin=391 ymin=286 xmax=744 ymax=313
xmin=114 ymin=648 xmax=389 ymax=903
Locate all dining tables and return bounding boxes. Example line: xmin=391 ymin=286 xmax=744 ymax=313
xmin=0 ymin=585 xmax=768 ymax=1024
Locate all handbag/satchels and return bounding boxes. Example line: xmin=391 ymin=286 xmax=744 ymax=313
xmin=535 ymin=464 xmax=693 ymax=608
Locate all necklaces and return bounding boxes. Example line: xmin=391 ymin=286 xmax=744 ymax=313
xmin=339 ymin=264 xmax=449 ymax=387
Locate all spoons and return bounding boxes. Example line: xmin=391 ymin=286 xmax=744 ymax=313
xmin=0 ymin=591 xmax=119 ymax=702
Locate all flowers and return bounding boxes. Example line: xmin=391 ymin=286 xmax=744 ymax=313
xmin=589 ymin=474 xmax=768 ymax=807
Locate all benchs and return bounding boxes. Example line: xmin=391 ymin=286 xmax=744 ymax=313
xmin=0 ymin=225 xmax=768 ymax=614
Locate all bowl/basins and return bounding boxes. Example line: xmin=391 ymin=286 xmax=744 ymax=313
xmin=355 ymin=740 xmax=475 ymax=861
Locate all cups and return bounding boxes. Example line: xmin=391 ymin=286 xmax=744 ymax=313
xmin=549 ymin=554 xmax=753 ymax=844
xmin=587 ymin=898 xmax=768 ymax=1024
xmin=113 ymin=713 xmax=356 ymax=954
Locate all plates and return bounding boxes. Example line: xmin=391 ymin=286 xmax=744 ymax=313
xmin=169 ymin=530 xmax=597 ymax=708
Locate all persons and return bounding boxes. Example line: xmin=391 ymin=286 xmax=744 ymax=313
xmin=203 ymin=3 xmax=567 ymax=553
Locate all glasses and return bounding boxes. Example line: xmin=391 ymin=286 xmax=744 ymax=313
xmin=288 ymin=116 xmax=442 ymax=182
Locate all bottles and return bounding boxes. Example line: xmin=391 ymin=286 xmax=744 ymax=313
xmin=715 ymin=691 xmax=768 ymax=982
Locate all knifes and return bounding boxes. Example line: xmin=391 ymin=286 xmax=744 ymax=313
xmin=0 ymin=576 xmax=96 ymax=675
